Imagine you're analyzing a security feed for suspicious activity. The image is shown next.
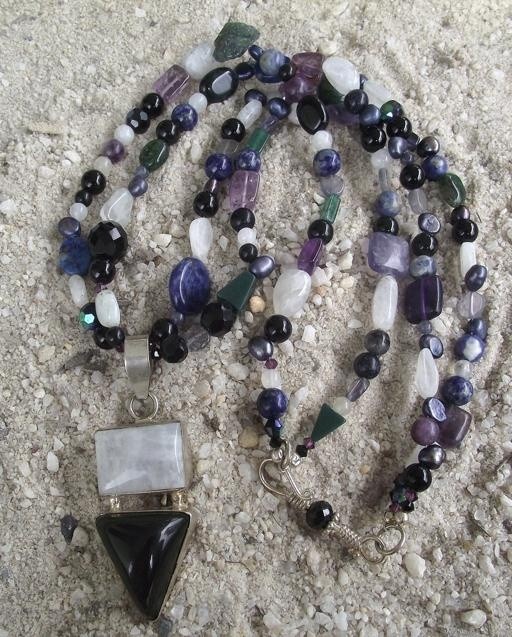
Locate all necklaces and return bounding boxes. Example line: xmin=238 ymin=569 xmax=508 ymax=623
xmin=50 ymin=21 xmax=494 ymax=622
